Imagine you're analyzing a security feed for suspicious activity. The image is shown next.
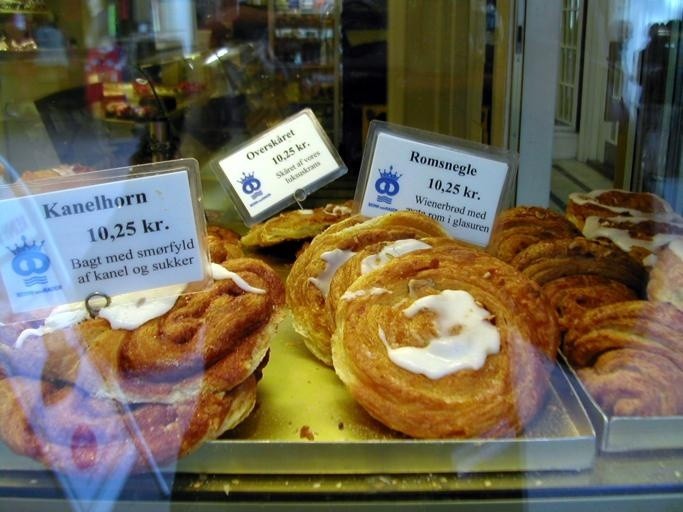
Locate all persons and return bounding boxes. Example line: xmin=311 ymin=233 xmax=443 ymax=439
xmin=603 ymin=19 xmax=635 ymax=192
xmin=206 ymin=2 xmax=252 ymax=128
xmin=630 ymin=22 xmax=668 ymax=194
xmin=657 ymin=20 xmax=682 ymax=200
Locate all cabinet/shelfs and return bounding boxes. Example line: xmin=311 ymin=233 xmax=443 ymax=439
xmin=268 ymin=0 xmax=344 ymax=154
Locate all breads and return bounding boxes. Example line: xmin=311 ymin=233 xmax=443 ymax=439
xmin=0 ymin=165 xmax=683 ymax=475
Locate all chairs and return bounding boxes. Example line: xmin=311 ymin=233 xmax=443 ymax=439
xmin=33 ymin=84 xmax=151 ymax=171
